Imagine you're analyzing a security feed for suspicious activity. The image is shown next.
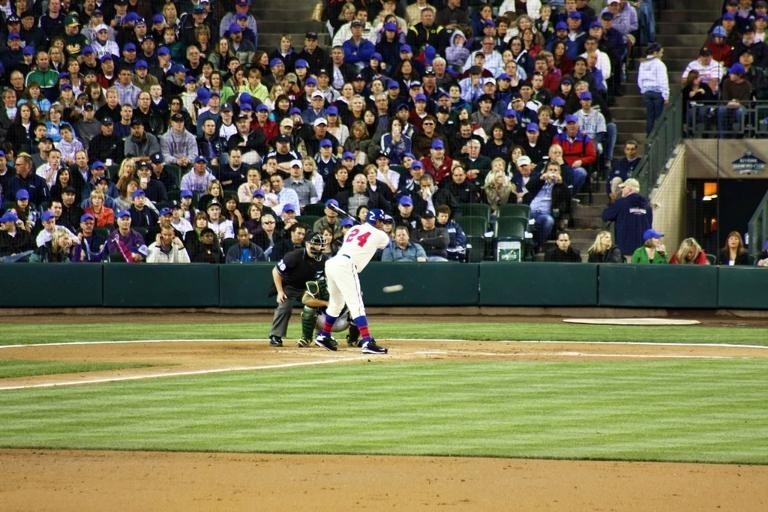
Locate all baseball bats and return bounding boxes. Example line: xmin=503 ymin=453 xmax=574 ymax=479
xmin=328 ymin=203 xmax=360 ymax=224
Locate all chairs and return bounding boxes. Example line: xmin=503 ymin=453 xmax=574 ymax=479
xmin=93 ymin=144 xmax=601 ymax=261
xmin=703 ymin=253 xmax=717 ymax=265
xmin=745 ymin=253 xmax=757 ymax=266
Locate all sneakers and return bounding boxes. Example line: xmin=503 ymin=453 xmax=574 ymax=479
xmin=297 ymin=336 xmax=313 ymax=347
xmin=270 ymin=335 xmax=282 ymax=347
xmin=361 ymin=339 xmax=388 ymax=354
xmin=346 ymin=340 xmax=362 ymax=351
xmin=314 ymin=334 xmax=337 ymax=352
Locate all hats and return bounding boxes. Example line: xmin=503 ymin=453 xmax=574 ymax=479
xmin=726 ymin=63 xmax=744 ymax=73
xmin=618 ymin=178 xmax=640 ymax=188
xmin=2 ymin=2 xmax=614 ymax=251
xmin=711 ymin=25 xmax=727 ymax=38
xmin=722 ymin=13 xmax=735 ymax=20
xmin=643 ymin=229 xmax=665 ymax=242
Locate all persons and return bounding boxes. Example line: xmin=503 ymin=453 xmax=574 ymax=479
xmin=270 ymin=209 xmax=388 ymax=353
xmin=0 ymin=0 xmax=768 ymax=267
